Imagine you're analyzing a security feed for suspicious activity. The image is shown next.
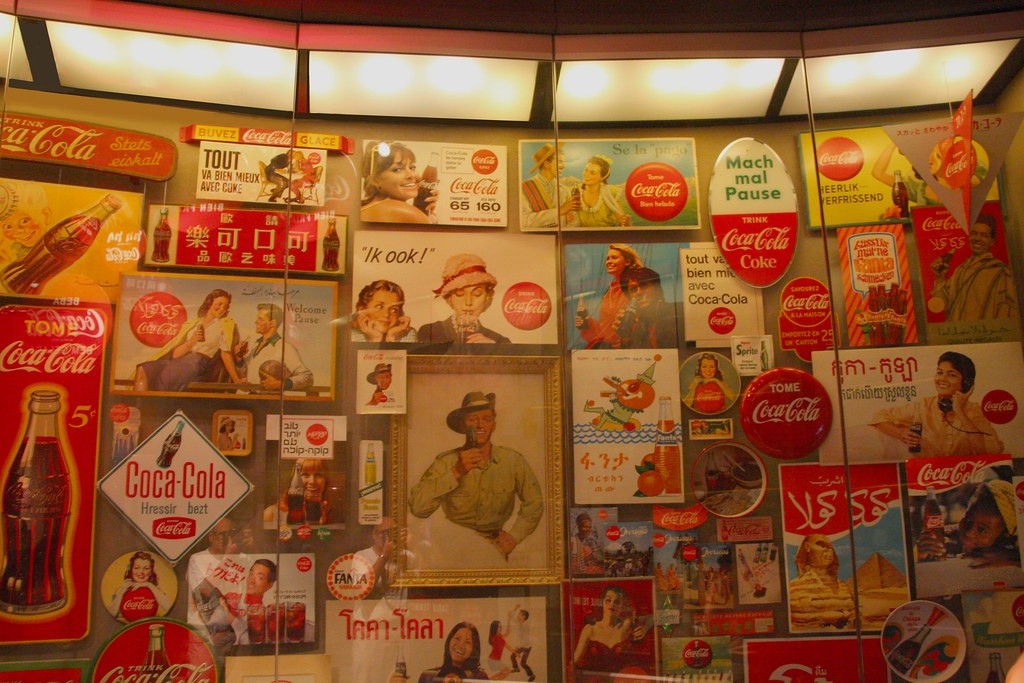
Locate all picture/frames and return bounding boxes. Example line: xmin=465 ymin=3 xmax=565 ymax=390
xmin=389 ymin=353 xmax=567 ymax=588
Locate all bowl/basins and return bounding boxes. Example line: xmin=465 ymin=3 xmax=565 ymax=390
xmin=731 ymin=461 xmax=762 ymax=487
xmin=690 ymin=440 xmax=769 ymax=520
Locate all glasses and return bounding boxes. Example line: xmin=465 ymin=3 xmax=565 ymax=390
xmin=370 ymin=141 xmax=397 ymax=175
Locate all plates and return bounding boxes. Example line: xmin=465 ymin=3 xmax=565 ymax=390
xmin=880 ymin=600 xmax=967 ymax=683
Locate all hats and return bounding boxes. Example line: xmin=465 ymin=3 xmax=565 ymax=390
xmin=447 ymin=391 xmax=495 ymax=434
xmin=958 ymin=480 xmax=1017 ymax=537
xmin=529 ymin=142 xmax=563 ymax=173
xmin=433 ymin=253 xmax=497 ymax=298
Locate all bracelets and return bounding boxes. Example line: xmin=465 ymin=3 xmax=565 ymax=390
xmin=379 ymin=553 xmax=387 ymax=560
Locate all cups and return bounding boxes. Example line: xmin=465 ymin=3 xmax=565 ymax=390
xmin=246 ymin=603 xmax=266 ymax=644
xmin=265 ymin=603 xmax=286 ymax=643
xmin=719 ymin=466 xmax=731 ymax=490
xmin=305 ymin=488 xmax=322 ymax=523
xmin=285 ymin=602 xmax=306 ymax=643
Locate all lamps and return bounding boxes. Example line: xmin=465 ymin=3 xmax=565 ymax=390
xmin=0 ymin=0 xmax=1024 ymax=132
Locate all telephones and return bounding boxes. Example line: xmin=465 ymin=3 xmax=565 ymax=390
xmin=939 ymin=379 xmax=974 ymax=412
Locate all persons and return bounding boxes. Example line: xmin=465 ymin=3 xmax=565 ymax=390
xmin=264 ymin=458 xmax=341 ymax=530
xmin=791 ymin=534 xmax=854 ymax=610
xmin=575 ymin=242 xmax=684 ymax=349
xmin=389 ymin=621 xmax=490 ymax=683
xmin=913 ymin=478 xmax=1021 ymax=566
xmin=219 ymin=417 xmax=238 ymax=450
xmin=227 ymin=558 xmax=278 ymax=645
xmin=353 ymin=516 xmax=417 ymax=683
xmin=186 ymin=517 xmax=258 ymax=656
xmin=361 ymin=141 xmax=439 ymax=224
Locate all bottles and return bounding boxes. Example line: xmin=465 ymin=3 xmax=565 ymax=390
xmin=760 ymin=341 xmax=769 ymax=371
xmin=923 ymin=486 xmax=944 ymax=561
xmin=887 ymin=624 xmax=931 ymax=674
xmin=151 ymin=208 xmax=172 ymax=262
xmin=933 ymin=247 xmax=956 ymax=273
xmin=571 ymin=182 xmax=581 ymax=211
xmin=694 ymin=641 xmax=699 ymax=664
xmin=654 ymin=397 xmax=681 ymax=497
xmin=892 ymin=170 xmax=908 ymax=217
xmin=458 ymin=310 xmax=477 ymax=343
xmin=631 ymin=611 xmax=642 ymax=642
xmin=577 ymin=294 xmax=588 ymax=330
xmin=321 ymin=218 xmax=340 ymax=271
xmin=197 ymin=319 xmax=205 ymax=342
xmin=287 ymin=463 xmax=305 ymax=525
xmin=986 ymin=652 xmax=1005 ymax=683
xmin=1 ymin=194 xmax=124 ymax=295
xmin=395 ymin=644 xmax=406 ymax=676
xmin=237 ymin=335 xmax=251 ymax=358
xmin=464 ymin=425 xmax=477 ymax=450
xmin=1 ymin=390 xmax=73 ymax=615
xmin=705 ymin=451 xmax=720 ymax=491
xmin=139 ymin=624 xmax=172 ymax=683
xmin=739 ymin=543 xmax=777 ymax=565
xmin=156 ymin=421 xmax=185 ymax=468
xmin=413 ymin=151 xmax=439 ymax=216
xmin=617 ymin=297 xmax=638 ymax=339
xmin=868 ymin=282 xmax=908 ymax=345
xmin=908 ymin=404 xmax=923 ymax=453
xmin=365 ymin=443 xmax=376 ymax=485
